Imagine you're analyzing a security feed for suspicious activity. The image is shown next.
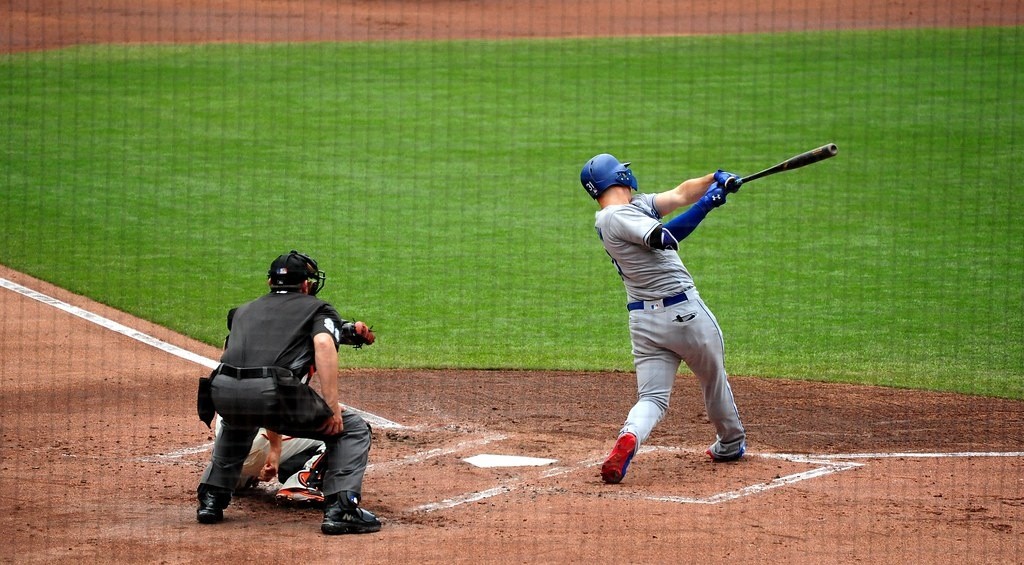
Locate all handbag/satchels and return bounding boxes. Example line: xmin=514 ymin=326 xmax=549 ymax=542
xmin=274 ymin=367 xmax=333 ymax=436
xmin=197 ymin=364 xmax=221 ymax=429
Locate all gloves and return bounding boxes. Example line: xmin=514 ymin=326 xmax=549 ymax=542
xmin=714 ymin=169 xmax=743 ymax=193
xmin=700 ymin=181 xmax=730 ymax=211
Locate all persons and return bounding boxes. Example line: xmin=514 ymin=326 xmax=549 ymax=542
xmin=580 ymin=152 xmax=745 ymax=483
xmin=196 ymin=251 xmax=381 ymax=535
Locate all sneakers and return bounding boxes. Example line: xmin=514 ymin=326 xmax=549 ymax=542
xmin=321 ymin=491 xmax=382 ymax=532
xmin=276 ymin=487 xmax=326 ymax=509
xmin=197 ymin=484 xmax=227 ymax=522
xmin=601 ymin=432 xmax=638 ymax=483
xmin=706 ymin=445 xmax=746 ymax=462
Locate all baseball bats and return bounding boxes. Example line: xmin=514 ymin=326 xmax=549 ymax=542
xmin=737 ymin=143 xmax=837 ymax=180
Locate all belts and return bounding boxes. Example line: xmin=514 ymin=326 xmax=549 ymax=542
xmin=628 ymin=294 xmax=687 ymax=312
xmin=220 ymin=365 xmax=291 ymax=379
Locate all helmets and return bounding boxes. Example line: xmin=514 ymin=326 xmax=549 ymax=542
xmin=269 ymin=251 xmax=326 ymax=295
xmin=580 ymin=153 xmax=638 ymax=199
